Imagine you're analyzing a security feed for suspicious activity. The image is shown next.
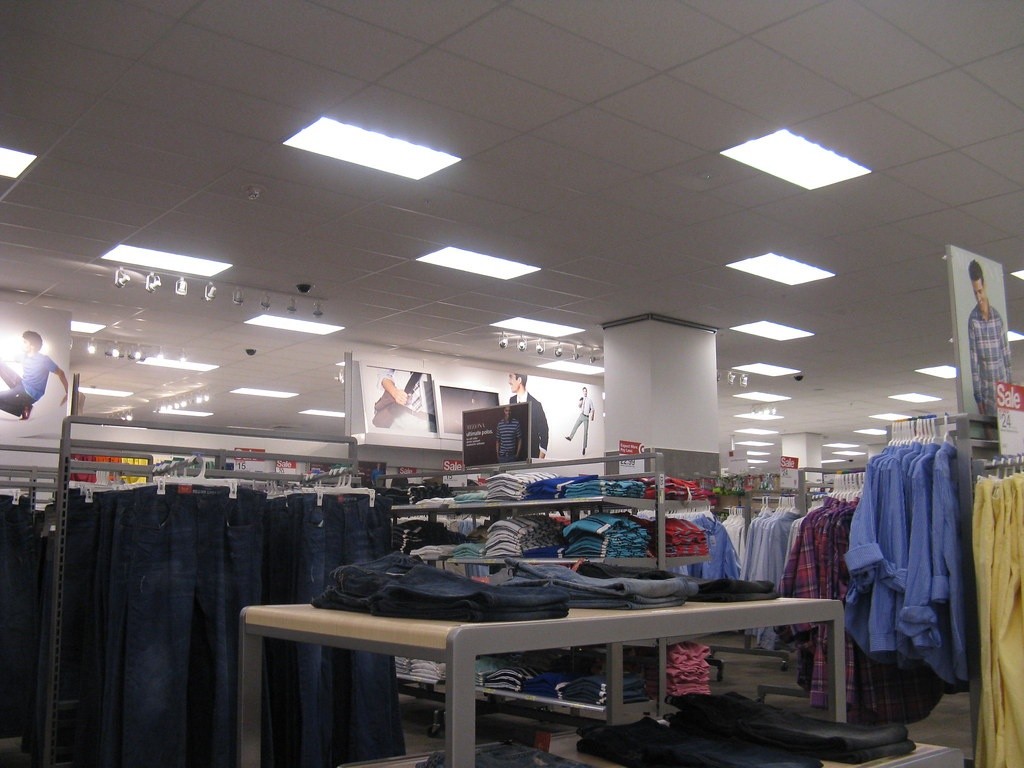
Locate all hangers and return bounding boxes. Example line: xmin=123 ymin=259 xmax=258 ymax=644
xmin=992 ymin=459 xmax=1000 ymax=479
xmin=887 ymin=415 xmax=955 ymax=447
xmin=1001 ymin=458 xmax=1008 ymax=478
xmin=76 ymin=455 xmax=240 ymax=497
xmin=1016 ymin=456 xmax=1024 ymax=473
xmin=610 ymin=472 xmax=865 ymax=526
xmin=254 ymin=464 xmax=375 ymax=508
xmin=1008 ymin=458 xmax=1016 ymax=474
xmin=0 ymin=487 xmax=21 ymax=505
xmin=1021 ymin=455 xmax=1024 ymax=463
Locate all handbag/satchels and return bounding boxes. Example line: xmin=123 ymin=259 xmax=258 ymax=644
xmin=372 ymin=389 xmax=400 ymax=428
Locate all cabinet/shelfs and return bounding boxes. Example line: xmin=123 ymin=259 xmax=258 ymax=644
xmin=376 ymin=452 xmax=713 ymax=737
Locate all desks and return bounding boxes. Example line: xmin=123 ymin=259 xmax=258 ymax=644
xmin=239 ymin=596 xmax=847 ymax=768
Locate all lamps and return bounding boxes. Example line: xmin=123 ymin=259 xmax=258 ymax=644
xmin=740 ymin=374 xmax=747 ymax=387
xmin=70 ymin=337 xmax=210 ymax=420
xmin=536 ymin=338 xmax=545 ymax=355
xmin=286 ymin=296 xmax=298 ymax=315
xmin=231 ymin=288 xmax=248 ymax=304
xmin=114 ymin=266 xmax=131 ymax=289
xmin=750 ymin=402 xmax=776 ymax=415
xmin=145 ymin=272 xmax=162 ymax=293
xmin=204 ymin=282 xmax=217 ymax=303
xmin=499 ymin=330 xmax=509 ymax=349
xmin=570 ymin=344 xmax=583 ymax=361
xmin=589 ymin=348 xmax=601 ymax=364
xmin=727 ymin=372 xmax=736 ymax=386
xmin=312 ymin=299 xmax=324 ymax=318
xmin=175 ymin=277 xmax=188 ymax=296
xmin=259 ymin=293 xmax=272 ymax=311
xmin=717 ymin=368 xmax=720 ymax=383
xmin=554 ymin=341 xmax=564 ymax=357
xmin=516 ymin=333 xmax=528 ymax=352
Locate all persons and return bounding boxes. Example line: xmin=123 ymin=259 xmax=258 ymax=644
xmin=377 ymin=366 xmax=433 ymax=433
xmin=508 ymin=373 xmax=549 ymax=459
xmin=565 ymin=387 xmax=596 ymax=455
xmin=968 ymin=260 xmax=1012 ymax=417
xmin=495 ymin=407 xmax=522 ymax=462
xmin=0 ymin=329 xmax=69 ymax=420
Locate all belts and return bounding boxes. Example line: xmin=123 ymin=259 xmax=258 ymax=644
xmin=582 ymin=413 xmax=589 ymax=417
xmin=406 ymin=407 xmax=435 ymax=422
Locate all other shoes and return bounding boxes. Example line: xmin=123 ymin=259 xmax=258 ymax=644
xmin=582 ymin=449 xmax=585 ymax=455
xmin=565 ymin=437 xmax=571 ymax=441
xmin=19 ymin=405 xmax=33 ymax=420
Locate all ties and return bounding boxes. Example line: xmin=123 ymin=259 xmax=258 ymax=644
xmin=412 ymin=380 xmax=423 ymax=413
